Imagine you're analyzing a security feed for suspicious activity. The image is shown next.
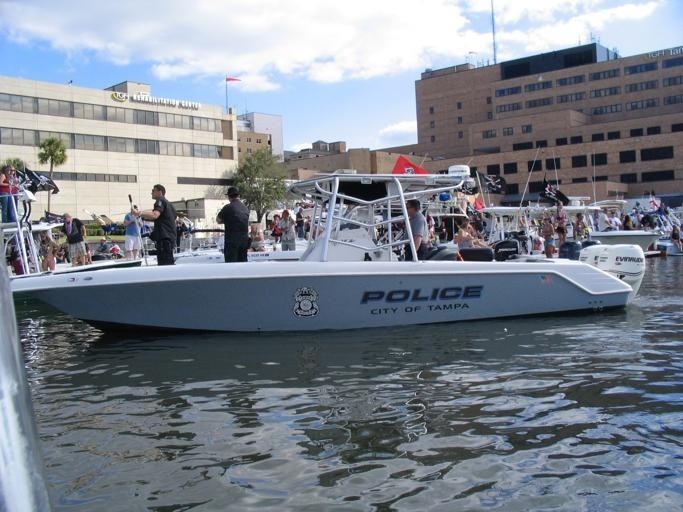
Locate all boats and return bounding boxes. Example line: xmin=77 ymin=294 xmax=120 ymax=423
xmin=466 ymin=148 xmax=660 ymax=301
xmin=6 ymin=175 xmax=143 ymax=280
xmin=9 ymin=173 xmax=661 ymax=333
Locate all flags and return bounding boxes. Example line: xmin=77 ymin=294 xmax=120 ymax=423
xmin=14 ymin=169 xmax=44 ymax=192
xmin=458 ymin=186 xmax=477 ymax=196
xmin=477 ymin=171 xmax=506 ymax=192
xmin=24 ymin=168 xmax=59 ymax=196
xmin=226 ymin=77 xmax=242 ymax=82
xmin=539 ymin=178 xmax=570 ymax=206
xmin=392 ymin=155 xmax=429 ymax=174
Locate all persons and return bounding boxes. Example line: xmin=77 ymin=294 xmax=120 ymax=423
xmin=426 ymin=201 xmax=683 ymax=259
xmin=178 ymin=211 xmax=189 ymax=231
xmin=91 ymin=239 xmax=124 ymax=261
xmin=4 ymin=212 xmax=93 ymax=272
xmin=0 ymin=163 xmax=27 ymax=223
xmin=401 ymin=200 xmax=429 ymax=261
xmin=215 ymin=187 xmax=250 ymax=263
xmin=129 ymin=183 xmax=176 ymax=265
xmin=248 ymin=202 xmax=324 ymax=252
xmin=124 ymin=204 xmax=143 ymax=260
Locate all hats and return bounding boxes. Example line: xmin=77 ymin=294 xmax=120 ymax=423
xmin=225 ymin=187 xmax=242 ymax=195
